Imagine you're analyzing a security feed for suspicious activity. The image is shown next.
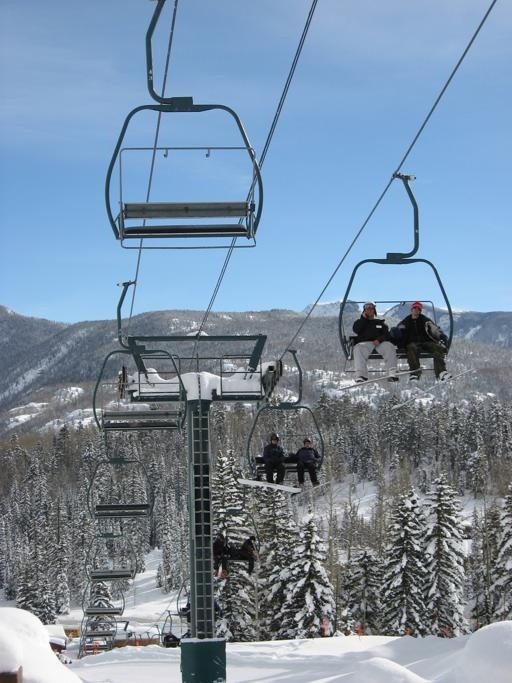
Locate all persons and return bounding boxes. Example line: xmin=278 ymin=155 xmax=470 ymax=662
xmin=397 ymin=302 xmax=452 ymax=381
xmin=353 ymin=301 xmax=398 ymax=382
xmin=213 ymin=533 xmax=256 ymax=575
xmin=295 ymin=439 xmax=321 ymax=487
xmin=263 ymin=433 xmax=285 ymax=484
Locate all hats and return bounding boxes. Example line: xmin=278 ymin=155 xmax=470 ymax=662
xmin=363 ymin=301 xmax=375 ymax=309
xmin=411 ymin=302 xmax=423 ymax=309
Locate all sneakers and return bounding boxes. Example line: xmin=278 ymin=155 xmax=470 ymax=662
xmin=411 ymin=374 xmax=419 ymax=381
xmin=313 ymin=480 xmax=320 ymax=486
xmin=355 ymin=376 xmax=368 ymax=384
xmin=387 ymin=374 xmax=399 ymax=381
xmin=438 ymin=372 xmax=452 ymax=380
xmin=268 ymin=477 xmax=281 ymax=484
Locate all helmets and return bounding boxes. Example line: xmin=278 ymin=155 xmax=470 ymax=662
xmin=270 ymin=433 xmax=279 ymax=441
xmin=303 ymin=438 xmax=311 ymax=442
